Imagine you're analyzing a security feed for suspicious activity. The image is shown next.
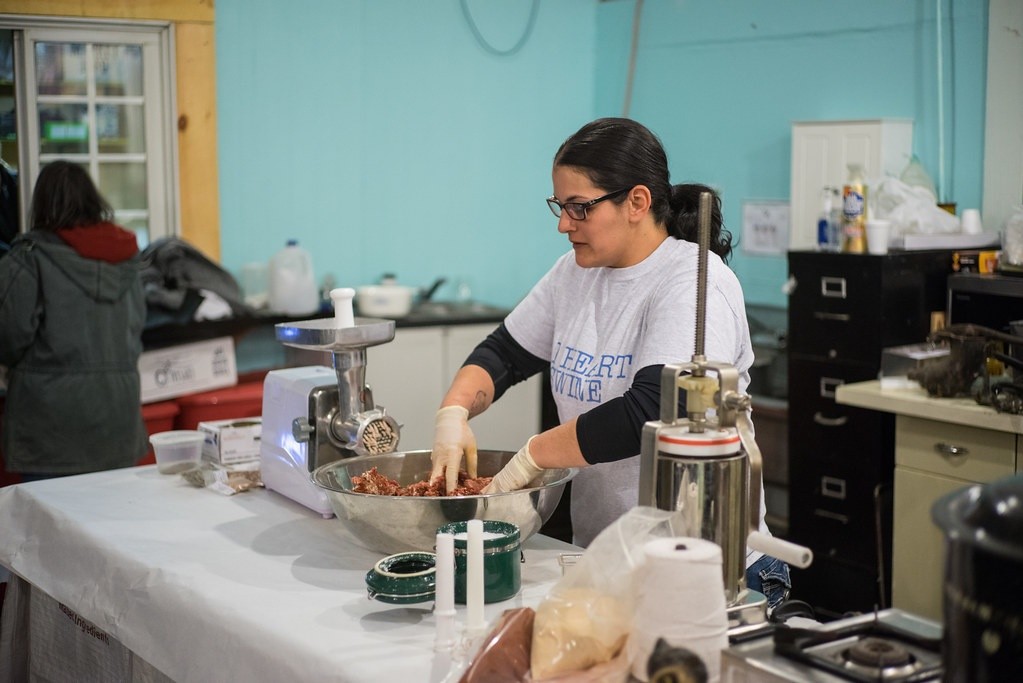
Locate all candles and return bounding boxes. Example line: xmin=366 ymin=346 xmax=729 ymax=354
xmin=435 ymin=532 xmax=455 ymax=611
xmin=465 ymin=518 xmax=484 ymax=627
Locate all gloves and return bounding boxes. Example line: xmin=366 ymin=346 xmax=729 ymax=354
xmin=429 ymin=405 xmax=478 ymax=497
xmin=478 ymin=435 xmax=545 ymax=496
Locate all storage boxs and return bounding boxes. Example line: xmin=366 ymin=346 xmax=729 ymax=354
xmin=889 ymin=231 xmax=999 ymax=251
xmin=881 ymin=342 xmax=951 ymax=400
xmin=197 ymin=416 xmax=263 ymax=464
xmin=790 ymin=118 xmax=914 ymax=252
xmin=174 ymin=369 xmax=268 ymax=430
xmin=136 ymin=335 xmax=239 ymax=406
xmin=133 ymin=399 xmax=181 ymax=465
xmin=953 ymin=250 xmax=1007 ymax=274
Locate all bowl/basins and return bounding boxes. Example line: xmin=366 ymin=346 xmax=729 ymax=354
xmin=436 ymin=520 xmax=524 ymax=605
xmin=355 ymin=284 xmax=413 ymax=317
xmin=308 ymin=447 xmax=579 ymax=553
xmin=148 ymin=430 xmax=205 ymax=475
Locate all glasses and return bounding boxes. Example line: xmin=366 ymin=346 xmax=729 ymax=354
xmin=545 ymin=188 xmax=631 ymax=222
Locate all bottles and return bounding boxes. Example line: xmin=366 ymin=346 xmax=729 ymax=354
xmin=381 ymin=274 xmax=398 ymax=285
xmin=816 ymin=185 xmax=845 ymax=252
xmin=264 ymin=238 xmax=321 ymax=317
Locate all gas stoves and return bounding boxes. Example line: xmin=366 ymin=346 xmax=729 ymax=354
xmin=719 ymin=606 xmax=946 ymax=682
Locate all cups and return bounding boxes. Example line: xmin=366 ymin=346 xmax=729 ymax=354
xmin=961 ymin=209 xmax=982 ymax=235
xmin=864 ymin=219 xmax=891 ymax=256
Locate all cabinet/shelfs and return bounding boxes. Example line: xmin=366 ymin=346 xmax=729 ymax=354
xmin=365 ymin=322 xmax=545 ymax=454
xmin=786 ymin=244 xmax=1002 ymax=624
xmin=945 ymin=273 xmax=1023 ymax=388
xmin=892 ymin=414 xmax=1016 ymax=627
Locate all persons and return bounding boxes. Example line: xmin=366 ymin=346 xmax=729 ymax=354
xmin=429 ymin=118 xmax=791 ymax=607
xmin=0 ymin=159 xmax=152 ymax=482
